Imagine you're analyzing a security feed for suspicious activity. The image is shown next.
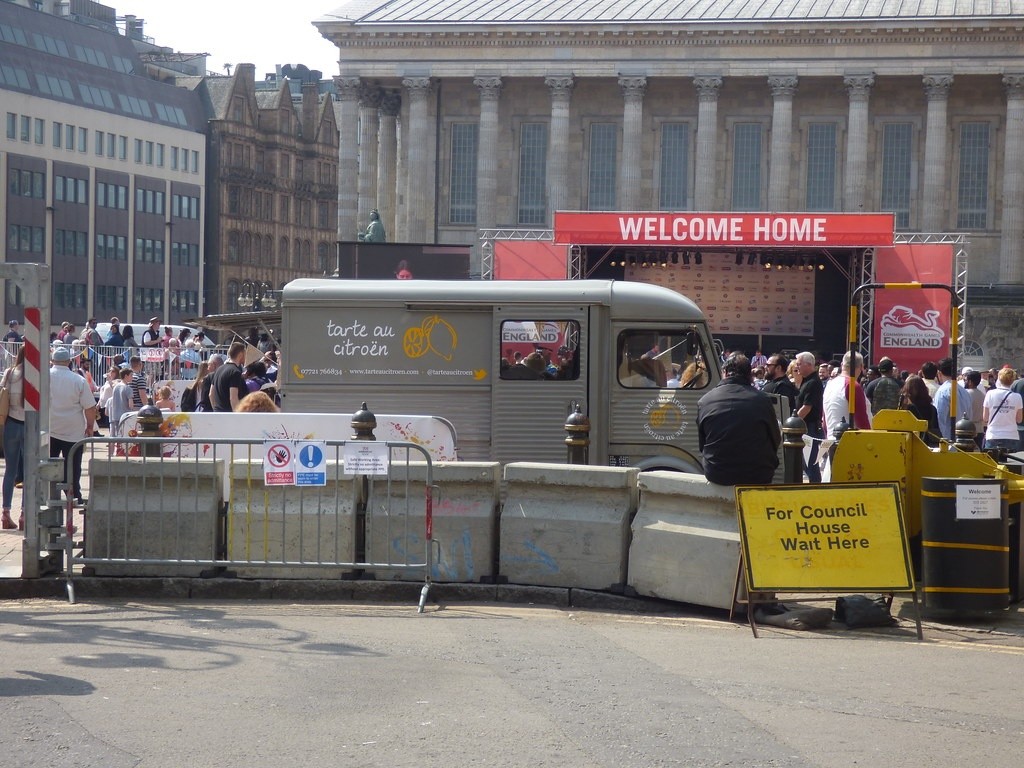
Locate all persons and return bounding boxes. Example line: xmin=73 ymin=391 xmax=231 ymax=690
xmin=49 ymin=317 xmax=281 ymax=437
xmin=641 ymin=345 xmax=705 ymax=386
xmin=502 ymin=346 xmax=573 ymax=378
xmin=750 ymin=350 xmax=1024 ymax=483
xmin=358 ymin=208 xmax=386 ymax=242
xmin=50 ymin=347 xmax=97 ymax=504
xmin=0 ymin=345 xmax=25 ymax=530
xmin=3 ymin=320 xmax=23 ymax=355
xmin=721 ymin=349 xmax=731 ymax=361
xmin=696 ymin=351 xmax=781 ymax=486
xmin=396 ymin=267 xmax=412 ymax=279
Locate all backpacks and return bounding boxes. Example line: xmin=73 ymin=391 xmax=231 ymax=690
xmin=181 ymin=379 xmax=203 ymax=412
xmin=249 ymin=376 xmax=277 ymax=401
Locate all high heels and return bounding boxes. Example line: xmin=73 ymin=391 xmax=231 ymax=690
xmin=18 ymin=511 xmax=24 ymax=531
xmin=1 ymin=510 xmax=18 ymax=529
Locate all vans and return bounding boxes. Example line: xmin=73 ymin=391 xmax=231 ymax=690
xmin=281 ymin=278 xmax=723 ymax=477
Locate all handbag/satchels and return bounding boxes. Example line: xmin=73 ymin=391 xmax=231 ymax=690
xmin=981 ymin=432 xmax=987 ymax=450
xmin=0 ymin=367 xmax=15 ymax=425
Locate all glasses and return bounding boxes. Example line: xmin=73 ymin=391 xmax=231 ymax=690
xmin=108 ymin=369 xmax=116 ymax=373
xmin=766 ymin=363 xmax=776 ymax=368
xmin=796 ymin=363 xmax=802 ymax=366
xmin=189 ymin=332 xmax=191 ymax=334
xmin=793 ymin=370 xmax=798 ymax=372
xmin=90 ymin=322 xmax=98 ymax=325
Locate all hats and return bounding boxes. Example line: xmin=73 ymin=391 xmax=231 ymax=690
xmin=879 ymin=359 xmax=894 ymax=372
xmin=962 ymin=366 xmax=973 ymax=375
xmin=148 ymin=317 xmax=163 ymax=328
xmin=52 ymin=347 xmax=70 ymax=361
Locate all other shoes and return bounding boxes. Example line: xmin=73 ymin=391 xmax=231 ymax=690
xmin=82 ymin=499 xmax=88 ymax=505
xmin=14 ymin=481 xmax=23 ymax=487
xmin=93 ymin=431 xmax=104 ymax=437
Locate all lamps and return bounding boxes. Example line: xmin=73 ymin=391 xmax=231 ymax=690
xmin=609 ymin=248 xmax=702 ymax=268
xmin=734 ymin=249 xmax=831 ymax=271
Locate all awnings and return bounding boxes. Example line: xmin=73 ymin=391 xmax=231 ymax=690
xmin=183 ymin=310 xmax=282 ymax=366
